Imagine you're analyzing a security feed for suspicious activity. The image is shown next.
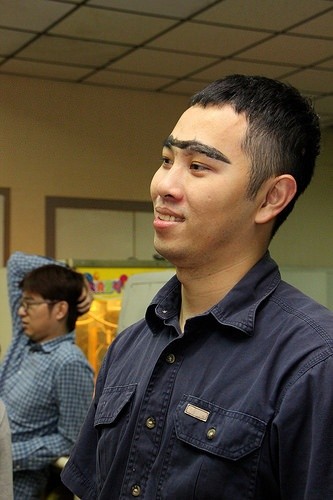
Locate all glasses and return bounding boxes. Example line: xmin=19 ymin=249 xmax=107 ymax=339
xmin=17 ymin=299 xmax=59 ymax=310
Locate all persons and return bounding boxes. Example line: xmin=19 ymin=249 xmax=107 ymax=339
xmin=1 ymin=251 xmax=98 ymax=500
xmin=57 ymin=73 xmax=332 ymax=499
